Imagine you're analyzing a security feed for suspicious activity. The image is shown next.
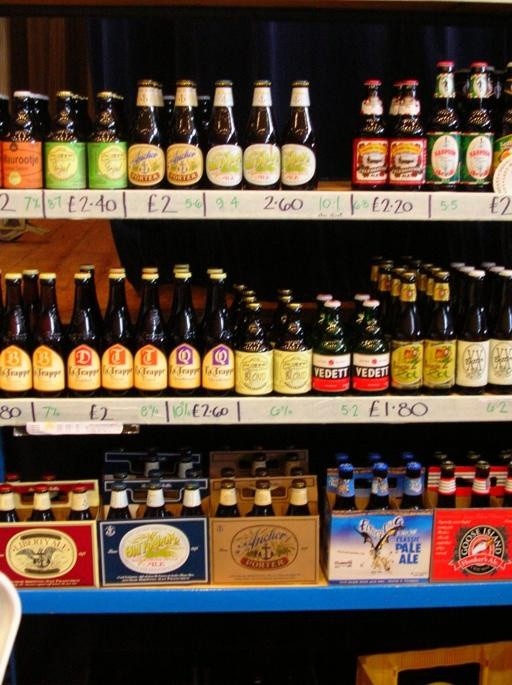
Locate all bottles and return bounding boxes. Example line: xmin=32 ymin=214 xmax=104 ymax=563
xmin=0 ymin=449 xmax=512 ymax=524
xmin=2 ymin=254 xmax=512 ymax=398
xmin=0 ymin=54 xmax=512 ymax=193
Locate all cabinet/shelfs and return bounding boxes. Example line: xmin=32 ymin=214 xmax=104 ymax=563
xmin=0 ymin=188 xmax=512 ymax=616
